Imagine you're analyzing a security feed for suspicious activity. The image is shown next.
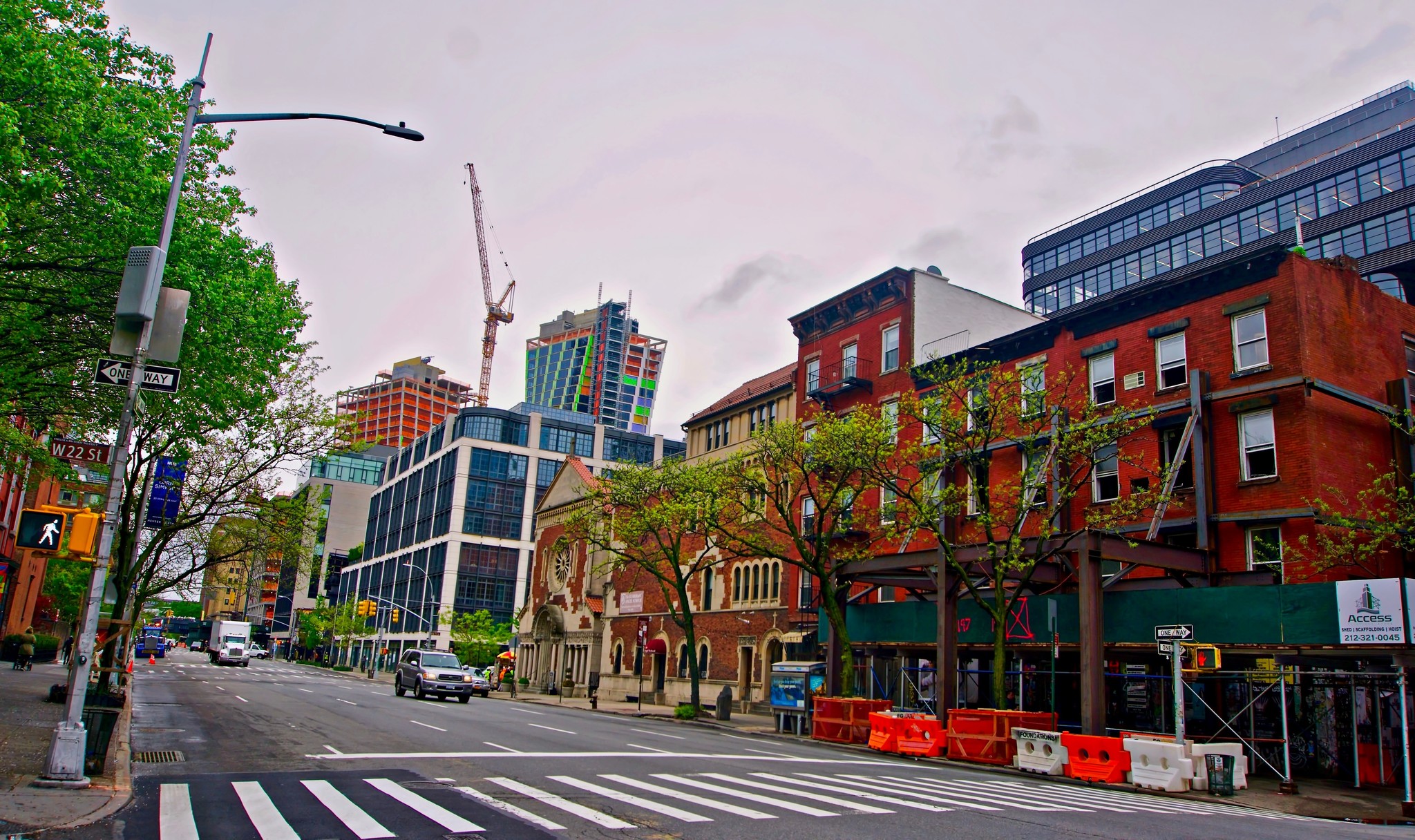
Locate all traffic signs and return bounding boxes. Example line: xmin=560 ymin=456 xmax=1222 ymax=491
xmin=1155 ymin=625 xmax=1194 ymax=640
xmin=1157 ymin=639 xmax=1188 ymax=658
xmin=94 ymin=358 xmax=182 ymax=394
xmin=424 ymin=642 xmax=436 ymax=648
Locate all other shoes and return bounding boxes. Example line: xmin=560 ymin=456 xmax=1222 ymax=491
xmin=66 ymin=664 xmax=68 ymax=666
xmin=497 ymin=690 xmax=499 ymax=692
xmin=63 ymin=660 xmax=66 ymax=665
xmin=361 ymin=672 xmax=365 ymax=673
xmin=20 ymin=667 xmax=25 ymax=671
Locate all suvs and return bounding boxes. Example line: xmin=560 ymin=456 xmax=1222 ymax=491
xmin=394 ymin=647 xmax=473 ymax=703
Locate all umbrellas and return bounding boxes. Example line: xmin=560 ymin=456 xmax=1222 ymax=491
xmin=497 ymin=651 xmax=517 ymax=666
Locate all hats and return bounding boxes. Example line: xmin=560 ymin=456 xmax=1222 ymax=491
xmin=486 ymin=666 xmax=490 ymax=669
xmin=510 ymin=667 xmax=513 ymax=670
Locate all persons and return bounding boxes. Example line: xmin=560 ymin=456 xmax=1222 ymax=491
xmin=484 ymin=667 xmax=491 ymax=682
xmin=166 ymin=641 xmax=171 ymax=652
xmin=311 ymin=650 xmax=316 ymax=661
xmin=920 ymin=661 xmax=937 ymax=698
xmin=323 ymin=652 xmax=329 ymax=668
xmin=361 ymin=655 xmax=367 ymax=673
xmin=203 ymin=640 xmax=208 ymax=653
xmin=18 ymin=626 xmax=37 ymax=671
xmin=62 ymin=636 xmax=74 ymax=665
xmin=497 ymin=666 xmax=514 ymax=692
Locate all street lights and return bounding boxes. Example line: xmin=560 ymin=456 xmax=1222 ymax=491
xmin=402 ymin=563 xmax=435 ymax=649
xmin=278 ymin=595 xmax=295 ymax=658
xmin=31 ymin=29 xmax=425 ymax=796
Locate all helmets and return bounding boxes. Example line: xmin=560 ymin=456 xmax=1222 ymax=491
xmin=69 ymin=636 xmax=74 ymax=639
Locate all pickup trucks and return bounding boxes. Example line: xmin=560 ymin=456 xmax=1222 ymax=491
xmin=248 ymin=643 xmax=269 ymax=660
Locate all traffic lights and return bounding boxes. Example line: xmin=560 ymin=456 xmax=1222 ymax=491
xmin=13 ymin=507 xmax=66 ymax=554
xmin=392 ymin=608 xmax=399 ymax=623
xmin=368 ymin=600 xmax=377 ymax=616
xmin=358 ymin=600 xmax=366 ymax=617
xmin=1192 ymin=646 xmax=1222 ymax=671
xmin=67 ymin=509 xmax=106 ymax=558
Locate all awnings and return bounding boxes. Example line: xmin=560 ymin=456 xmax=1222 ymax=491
xmin=644 ymin=639 xmax=666 ymax=654
xmin=780 ymin=628 xmax=818 ymax=642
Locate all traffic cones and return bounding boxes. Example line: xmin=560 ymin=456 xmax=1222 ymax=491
xmin=149 ymin=654 xmax=153 ymax=663
xmin=150 ymin=655 xmax=155 ymax=664
xmin=127 ymin=661 xmax=133 ymax=672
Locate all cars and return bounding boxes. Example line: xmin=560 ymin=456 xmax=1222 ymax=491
xmin=164 ymin=638 xmax=176 ymax=651
xmin=190 ymin=641 xmax=204 ymax=652
xmin=455 ymin=665 xmax=491 ymax=697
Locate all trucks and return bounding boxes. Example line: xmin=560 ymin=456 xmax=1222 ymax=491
xmin=135 ymin=618 xmax=166 ymax=658
xmin=208 ymin=620 xmax=252 ymax=667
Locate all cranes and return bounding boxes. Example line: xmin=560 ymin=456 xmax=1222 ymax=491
xmin=463 ymin=159 xmax=518 ymax=409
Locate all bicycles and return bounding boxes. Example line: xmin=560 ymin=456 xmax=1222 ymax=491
xmin=509 ymin=677 xmax=518 ymax=698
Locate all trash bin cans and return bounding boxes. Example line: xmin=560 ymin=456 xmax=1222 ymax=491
xmin=80 ymin=708 xmax=120 ymax=776
xmin=367 ymin=670 xmax=374 ymax=678
xmin=1204 ymin=754 xmax=1235 ymax=796
